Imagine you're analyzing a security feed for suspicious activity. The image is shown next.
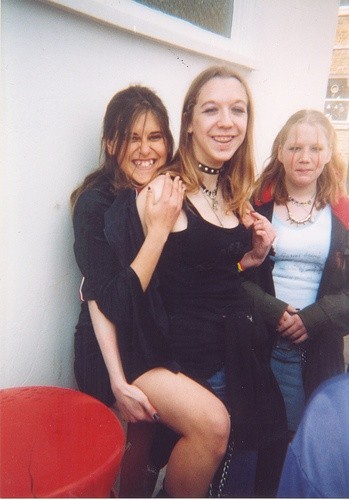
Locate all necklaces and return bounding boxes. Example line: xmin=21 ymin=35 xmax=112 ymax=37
xmin=190 ymin=157 xmax=227 ymax=230
xmin=282 ymin=187 xmax=320 ymax=226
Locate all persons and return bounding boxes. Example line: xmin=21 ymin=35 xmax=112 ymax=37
xmin=237 ymin=107 xmax=349 ymax=498
xmin=73 ymin=65 xmax=277 ymax=498
xmin=275 ymin=371 xmax=349 ymax=498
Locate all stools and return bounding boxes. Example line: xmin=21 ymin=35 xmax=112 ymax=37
xmin=0 ymin=380 xmax=125 ymax=498
